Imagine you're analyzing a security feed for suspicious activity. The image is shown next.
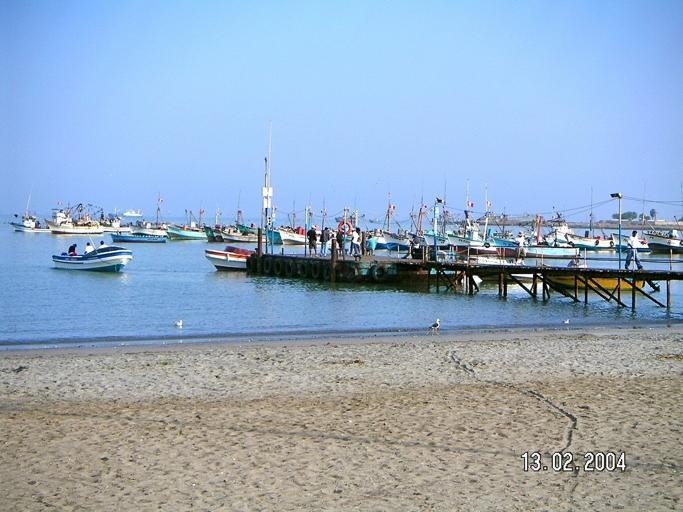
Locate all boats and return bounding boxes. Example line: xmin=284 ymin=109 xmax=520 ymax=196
xmin=8 ymin=197 xmax=269 ymax=242
xmin=52 ymin=244 xmax=133 ymax=273
xmin=204 ymin=246 xmax=255 ymax=271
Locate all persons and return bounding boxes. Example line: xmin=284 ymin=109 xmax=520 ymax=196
xmin=306 ymin=225 xmax=317 ymax=255
xmin=623 ymin=230 xmax=643 ymax=270
xmin=347 ymin=227 xmax=361 ymax=256
xmin=366 ymin=234 xmax=377 ymax=257
xmin=67 ymin=244 xmax=76 ymax=256
xmin=83 ymin=242 xmax=94 ymax=254
xmin=319 ymin=227 xmax=329 ymax=257
xmin=336 ymin=231 xmax=344 ymax=256
xmin=511 ymin=231 xmax=527 ymax=266
xmin=98 ymin=240 xmax=107 ymax=249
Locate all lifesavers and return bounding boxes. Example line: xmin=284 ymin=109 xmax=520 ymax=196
xmin=338 ymin=221 xmax=351 ymax=234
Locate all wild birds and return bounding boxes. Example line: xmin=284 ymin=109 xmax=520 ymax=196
xmin=563 ymin=318 xmax=571 ymax=324
xmin=428 ymin=318 xmax=441 ymax=336
xmin=175 ymin=319 xmax=183 ymax=327
